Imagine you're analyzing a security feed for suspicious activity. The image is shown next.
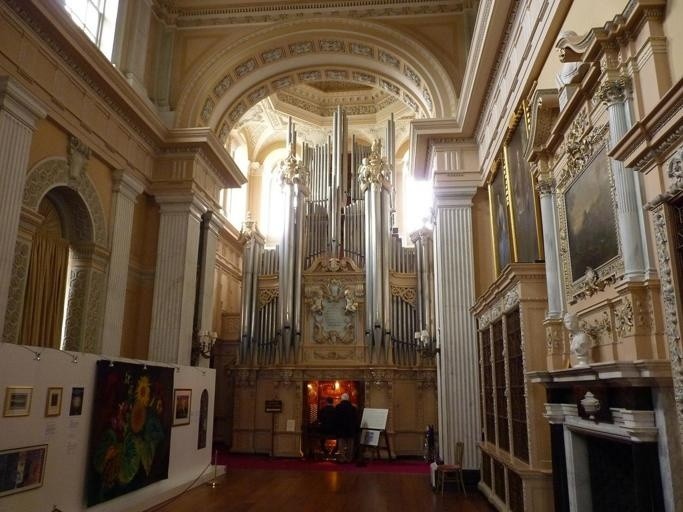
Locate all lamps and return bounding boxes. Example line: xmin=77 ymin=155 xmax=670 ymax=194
xmin=415 ymin=329 xmax=440 ymax=360
xmin=199 ymin=332 xmax=218 ymax=360
xmin=22 ymin=346 xmax=147 ymax=369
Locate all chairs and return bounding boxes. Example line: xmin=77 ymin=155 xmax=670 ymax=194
xmin=437 ymin=442 xmax=467 ymax=496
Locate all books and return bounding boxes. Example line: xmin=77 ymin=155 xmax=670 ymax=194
xmin=544 ymin=403 xmax=578 ymax=415
xmin=610 ymin=407 xmax=657 ymax=433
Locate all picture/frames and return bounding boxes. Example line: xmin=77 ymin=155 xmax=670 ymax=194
xmin=173 ymin=388 xmax=192 ymax=427
xmin=45 ymin=387 xmax=63 ymax=417
xmin=4 ymin=387 xmax=34 ymax=417
xmin=0 ymin=444 xmax=47 ymax=498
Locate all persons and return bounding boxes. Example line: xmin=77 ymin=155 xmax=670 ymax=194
xmin=344 ymin=290 xmax=356 ymax=312
xmin=562 ymin=312 xmax=593 ymax=367
xmin=318 ymin=394 xmax=358 ymax=462
xmin=310 ymin=291 xmax=323 ymax=312
